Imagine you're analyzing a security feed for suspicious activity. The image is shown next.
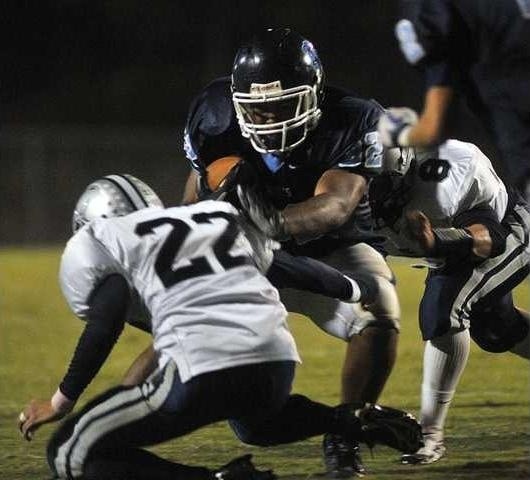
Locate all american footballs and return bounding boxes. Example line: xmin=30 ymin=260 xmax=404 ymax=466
xmin=203 ymin=155 xmax=244 ymax=193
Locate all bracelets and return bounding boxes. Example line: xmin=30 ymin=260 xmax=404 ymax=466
xmin=432 ymin=224 xmax=475 ymax=259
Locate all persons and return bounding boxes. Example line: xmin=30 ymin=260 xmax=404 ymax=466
xmin=119 ymin=25 xmax=421 ymax=479
xmin=16 ymin=171 xmax=424 ymax=478
xmin=346 ymin=101 xmax=528 ymax=464
xmin=377 ymin=0 xmax=530 ymax=195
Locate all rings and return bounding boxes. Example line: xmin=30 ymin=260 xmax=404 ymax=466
xmin=19 ymin=411 xmax=28 ymax=424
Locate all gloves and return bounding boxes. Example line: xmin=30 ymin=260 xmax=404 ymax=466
xmin=195 ymin=161 xmax=253 ymax=201
xmin=236 ymin=181 xmax=292 ymax=243
xmin=375 ymin=106 xmax=419 ymax=153
xmin=362 ymin=271 xmax=401 ymax=332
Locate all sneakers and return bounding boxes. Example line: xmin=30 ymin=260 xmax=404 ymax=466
xmin=399 ymin=419 xmax=447 ymax=465
xmin=219 ymin=454 xmax=278 ymax=479
xmin=337 ymin=401 xmax=425 ymax=454
xmin=322 ymin=433 xmax=367 ymax=478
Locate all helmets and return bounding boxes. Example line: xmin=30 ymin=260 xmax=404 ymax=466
xmin=230 ymin=26 xmax=330 ymax=155
xmin=354 ymin=147 xmax=416 ymax=222
xmin=71 ymin=171 xmax=164 ymax=235
